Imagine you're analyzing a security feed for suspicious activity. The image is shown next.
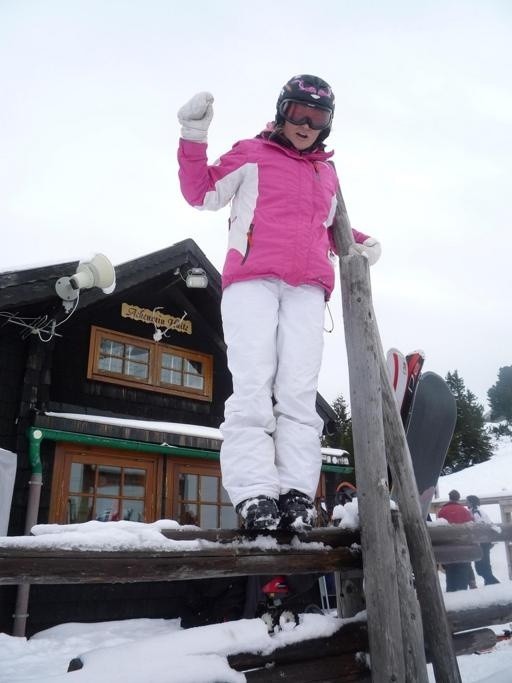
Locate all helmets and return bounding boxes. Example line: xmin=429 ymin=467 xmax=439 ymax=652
xmin=276 ymin=74 xmax=335 ymax=135
xmin=447 ymin=490 xmax=480 ymax=506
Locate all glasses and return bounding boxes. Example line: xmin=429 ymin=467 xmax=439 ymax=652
xmin=279 ymin=99 xmax=333 ymax=129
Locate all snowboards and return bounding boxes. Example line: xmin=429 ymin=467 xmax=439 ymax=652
xmin=390 ymin=370 xmax=457 ymax=527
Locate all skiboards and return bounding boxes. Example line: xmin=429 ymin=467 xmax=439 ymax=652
xmin=386 ymin=351 xmax=424 ymax=492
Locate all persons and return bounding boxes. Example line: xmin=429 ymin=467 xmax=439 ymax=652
xmin=177 ymin=74 xmax=382 ymax=530
xmin=437 ymin=490 xmax=500 ymax=592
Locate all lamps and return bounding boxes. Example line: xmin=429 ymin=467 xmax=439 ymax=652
xmin=54 ymin=253 xmax=115 ymax=301
xmin=185 ymin=267 xmax=209 ymax=289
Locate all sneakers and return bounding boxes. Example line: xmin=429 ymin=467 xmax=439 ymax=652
xmin=236 ymin=490 xmax=317 ymax=530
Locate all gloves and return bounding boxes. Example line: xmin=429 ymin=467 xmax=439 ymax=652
xmin=351 ymin=237 xmax=382 ymax=266
xmin=177 ymin=91 xmax=215 ymax=140
